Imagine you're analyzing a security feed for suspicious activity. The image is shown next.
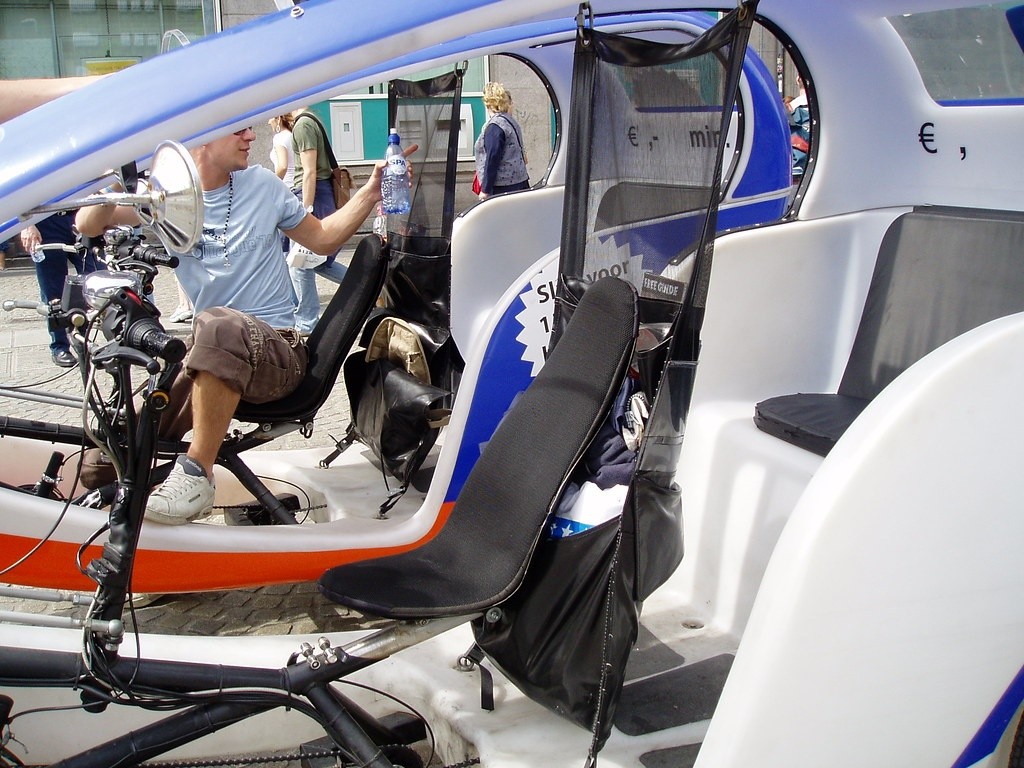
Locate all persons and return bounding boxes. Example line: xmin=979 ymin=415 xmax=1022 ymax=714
xmin=0 ymin=241 xmax=9 ymax=271
xmin=474 ymin=83 xmax=530 ymax=201
xmin=788 ymin=75 xmax=809 ymax=110
xmin=80 ymin=125 xmax=416 ymax=525
xmin=269 ymin=106 xmax=348 ymax=335
xmin=19 ymin=209 xmax=106 ymax=367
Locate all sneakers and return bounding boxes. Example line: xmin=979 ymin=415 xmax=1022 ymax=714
xmin=144 ymin=455 xmax=215 ymax=525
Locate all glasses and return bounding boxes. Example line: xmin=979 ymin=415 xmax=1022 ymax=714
xmin=233 ymin=126 xmax=252 ymax=135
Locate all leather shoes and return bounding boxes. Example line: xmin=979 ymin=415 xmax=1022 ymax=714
xmin=52 ymin=351 xmax=77 ymax=366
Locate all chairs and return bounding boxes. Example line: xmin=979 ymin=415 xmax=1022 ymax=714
xmin=317 ymin=273 xmax=641 ymax=621
xmin=233 ymin=232 xmax=389 ymax=425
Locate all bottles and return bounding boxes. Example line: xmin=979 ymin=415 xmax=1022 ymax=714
xmin=28 ymin=239 xmax=45 ymax=263
xmin=380 ymin=128 xmax=411 ymax=215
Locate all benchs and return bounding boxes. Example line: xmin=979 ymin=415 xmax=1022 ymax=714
xmin=750 ymin=197 xmax=1024 ymax=458
xmin=592 ymin=181 xmax=711 ymax=236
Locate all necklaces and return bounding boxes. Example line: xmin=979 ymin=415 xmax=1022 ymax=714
xmin=171 ymin=273 xmax=192 ymax=322
xmin=202 ymin=176 xmax=232 ymax=269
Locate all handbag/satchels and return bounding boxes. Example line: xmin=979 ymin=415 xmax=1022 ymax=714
xmin=331 ymin=166 xmax=356 ymax=210
xmin=471 ymin=172 xmax=482 ymax=195
xmin=285 ymin=243 xmax=327 ymax=270
